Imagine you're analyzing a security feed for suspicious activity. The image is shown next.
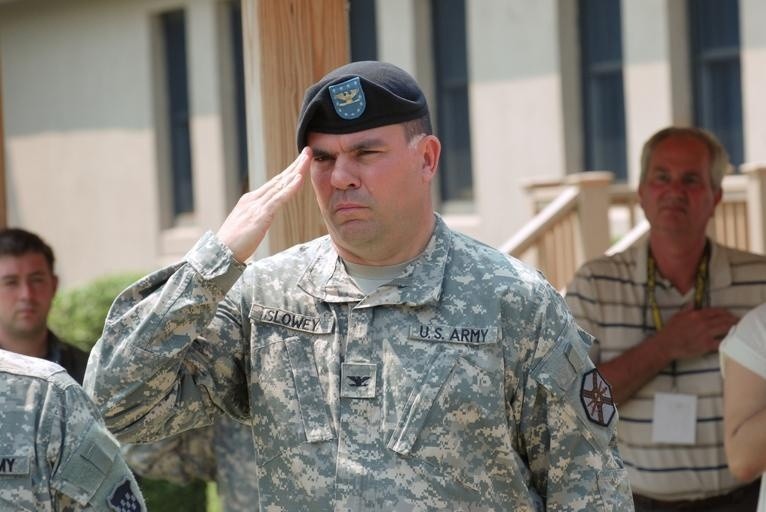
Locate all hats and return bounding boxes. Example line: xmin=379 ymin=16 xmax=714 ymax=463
xmin=296 ymin=61 xmax=429 ymax=156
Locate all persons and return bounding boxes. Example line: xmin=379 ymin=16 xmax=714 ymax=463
xmin=0 ymin=226 xmax=90 ymax=387
xmin=717 ymin=302 xmax=766 ymax=512
xmin=0 ymin=347 xmax=150 ymax=512
xmin=90 ymin=61 xmax=636 ymax=512
xmin=563 ymin=125 xmax=766 ymax=512
